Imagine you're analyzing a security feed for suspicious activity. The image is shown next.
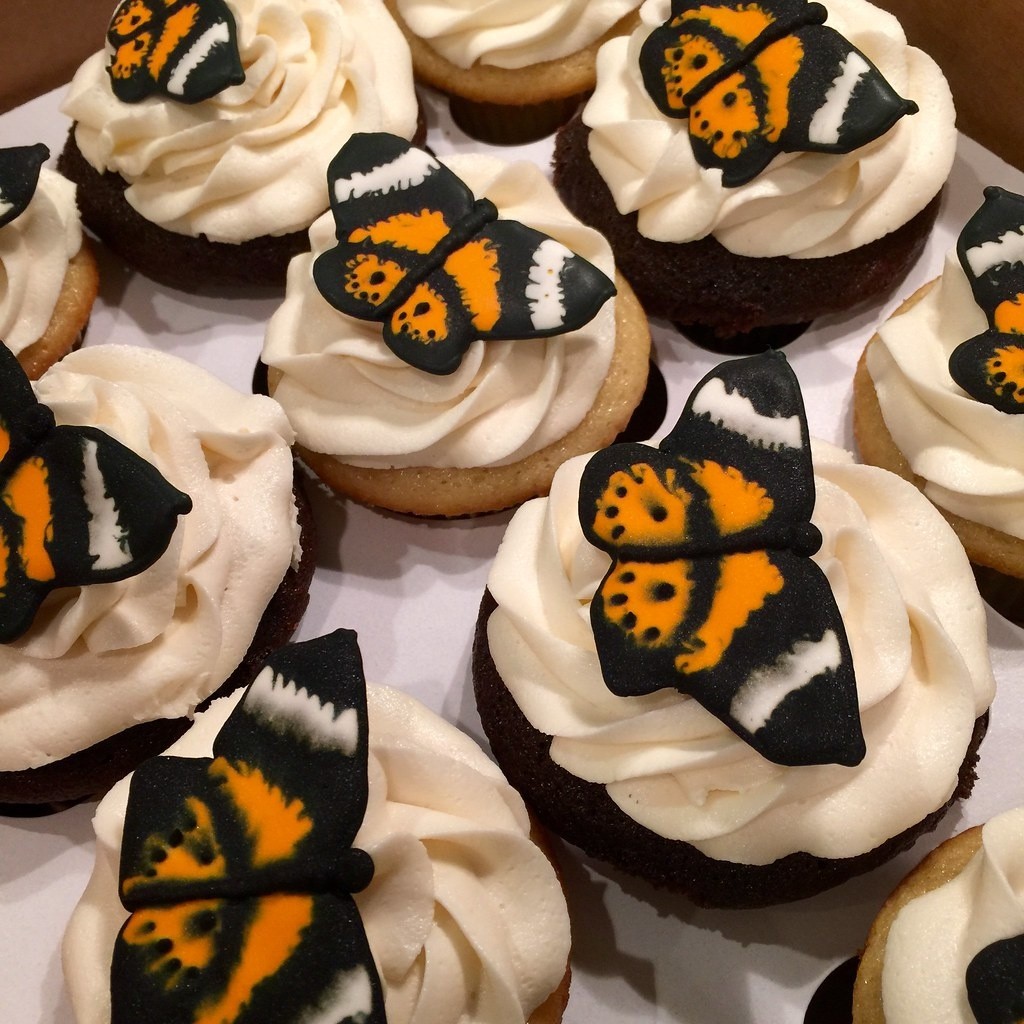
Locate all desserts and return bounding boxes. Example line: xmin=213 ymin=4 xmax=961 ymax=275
xmin=0 ymin=0 xmax=1024 ymax=1024
